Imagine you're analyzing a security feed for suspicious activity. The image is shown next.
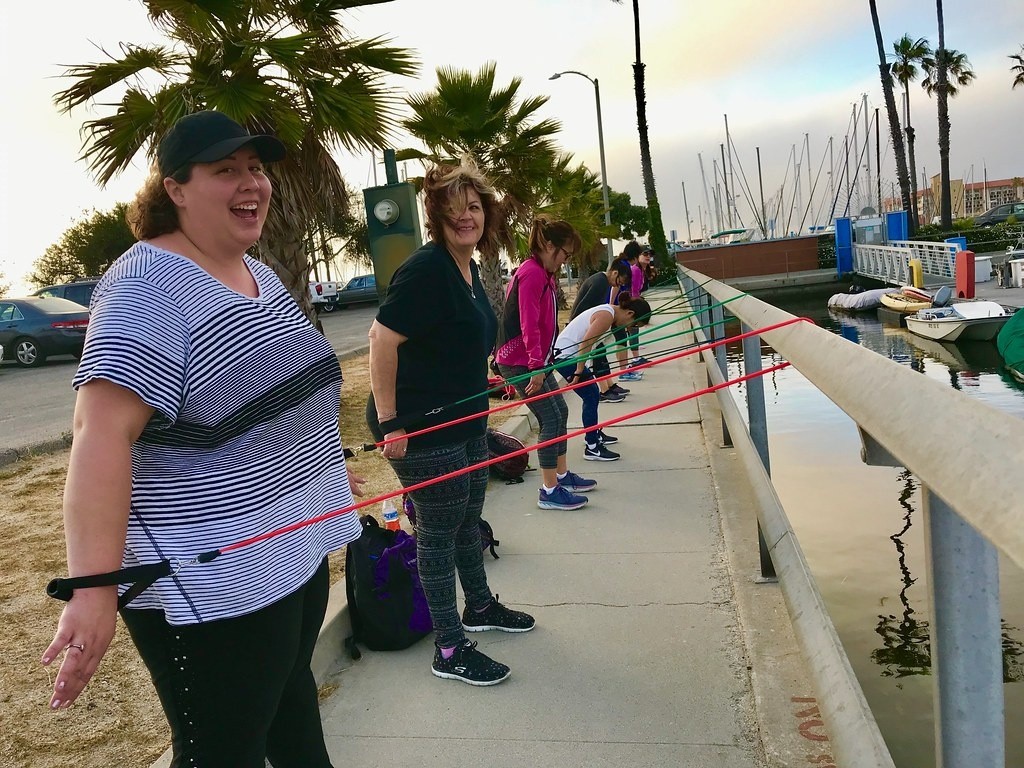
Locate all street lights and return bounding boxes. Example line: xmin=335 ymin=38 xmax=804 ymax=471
xmin=548 ymin=70 xmax=613 ymax=271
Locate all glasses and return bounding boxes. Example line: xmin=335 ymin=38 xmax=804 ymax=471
xmin=641 ymin=252 xmax=653 ymax=257
xmin=561 ymin=247 xmax=574 ymax=260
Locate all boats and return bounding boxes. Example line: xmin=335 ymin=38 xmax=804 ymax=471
xmin=827 ymin=288 xmax=902 ymax=311
xmin=996 ymin=307 xmax=1024 ymax=383
xmin=880 ymin=292 xmax=932 ymax=315
xmin=904 ymin=301 xmax=1015 ymax=342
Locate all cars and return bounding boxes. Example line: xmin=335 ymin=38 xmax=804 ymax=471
xmin=0 ymin=296 xmax=91 ymax=367
xmin=322 ymin=274 xmax=379 ymax=313
xmin=639 ymin=240 xmax=689 ymax=266
xmin=970 ymin=203 xmax=1024 ymax=230
xmin=29 ymin=275 xmax=105 ymax=310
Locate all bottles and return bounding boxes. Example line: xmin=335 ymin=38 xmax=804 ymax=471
xmin=382 ymin=498 xmax=400 ymax=532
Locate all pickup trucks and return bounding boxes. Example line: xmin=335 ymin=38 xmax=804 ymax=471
xmin=308 ymin=279 xmax=340 ymax=305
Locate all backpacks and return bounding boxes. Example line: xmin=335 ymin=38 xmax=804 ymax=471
xmin=402 ymin=490 xmax=500 ymax=559
xmin=345 ymin=515 xmax=434 ymax=661
xmin=486 ymin=426 xmax=537 ymax=485
xmin=488 ymin=375 xmax=516 ymax=402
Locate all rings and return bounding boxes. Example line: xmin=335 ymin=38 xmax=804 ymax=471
xmin=402 ymin=448 xmax=407 ymax=452
xmin=390 ymin=449 xmax=396 ymax=453
xmin=65 ymin=644 xmax=86 ymax=654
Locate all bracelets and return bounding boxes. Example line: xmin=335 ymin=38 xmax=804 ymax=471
xmin=378 ymin=410 xmax=398 ymax=421
xmin=573 ymin=373 xmax=582 ymax=377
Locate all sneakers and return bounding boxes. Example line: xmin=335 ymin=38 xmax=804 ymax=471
xmin=584 ymin=443 xmax=620 ymax=461
xmin=462 ymin=594 xmax=536 ymax=633
xmin=599 ymin=383 xmax=630 ymax=403
xmin=618 ymin=365 xmax=645 ymax=381
xmin=557 ymin=471 xmax=597 ymax=492
xmin=537 ymin=484 xmax=588 ymax=510
xmin=431 ymin=638 xmax=512 ymax=686
xmin=598 ymin=427 xmax=619 ymax=444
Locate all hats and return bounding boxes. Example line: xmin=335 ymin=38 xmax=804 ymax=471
xmin=157 ymin=111 xmax=287 ymax=177
xmin=641 ymin=247 xmax=653 ymax=252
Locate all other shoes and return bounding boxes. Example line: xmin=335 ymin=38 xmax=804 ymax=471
xmin=632 ymin=357 xmax=653 ymax=367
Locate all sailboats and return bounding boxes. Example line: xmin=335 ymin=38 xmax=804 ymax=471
xmin=682 ymin=90 xmax=993 ymax=250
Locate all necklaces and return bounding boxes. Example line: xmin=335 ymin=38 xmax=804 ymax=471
xmin=446 ymin=246 xmax=476 ymax=299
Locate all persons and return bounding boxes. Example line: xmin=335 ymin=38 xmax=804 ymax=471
xmin=568 ymin=257 xmax=633 ymax=403
xmin=370 ymin=165 xmax=536 ymax=685
xmin=552 ymin=291 xmax=653 ymax=461
xmin=42 ymin=106 xmax=366 ymax=768
xmin=629 ymin=245 xmax=655 ymax=368
xmin=493 ymin=219 xmax=599 ymax=511
xmin=605 ymin=241 xmax=644 ymax=381
